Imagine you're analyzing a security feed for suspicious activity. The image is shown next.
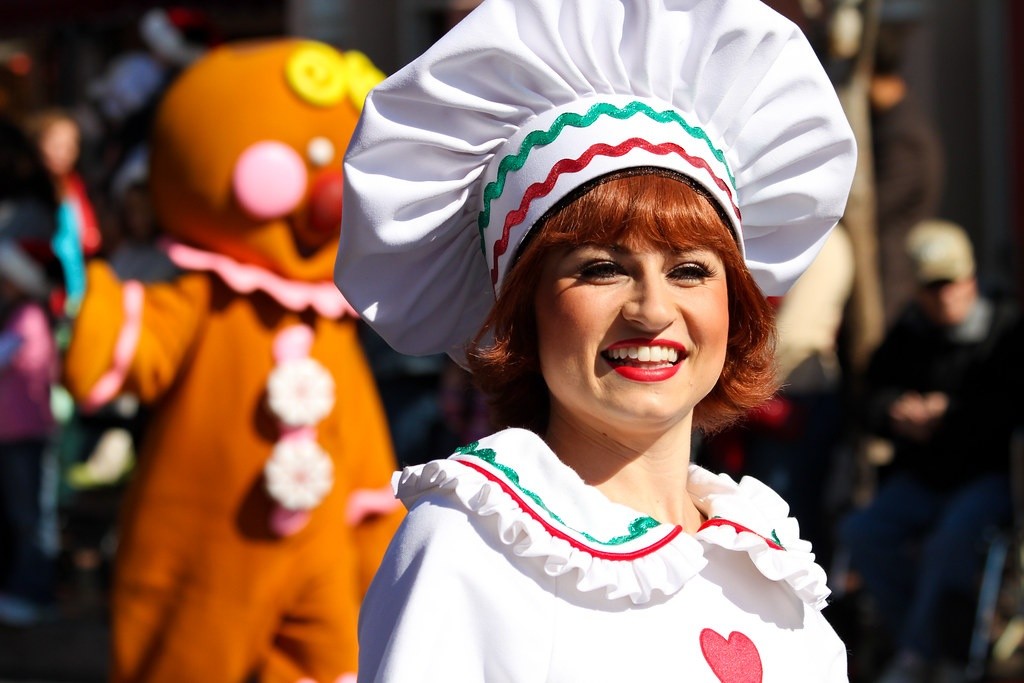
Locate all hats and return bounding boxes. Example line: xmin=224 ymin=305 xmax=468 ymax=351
xmin=902 ymin=219 xmax=974 ymax=286
xmin=333 ymin=0 xmax=858 ymax=375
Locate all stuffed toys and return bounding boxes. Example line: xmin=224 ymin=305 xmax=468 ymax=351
xmin=69 ymin=39 xmax=410 ymax=683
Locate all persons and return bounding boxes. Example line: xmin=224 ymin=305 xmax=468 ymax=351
xmin=334 ymin=0 xmax=856 ymax=683
xmin=0 ymin=0 xmax=213 ymax=625
xmin=684 ymin=46 xmax=1024 ymax=682
